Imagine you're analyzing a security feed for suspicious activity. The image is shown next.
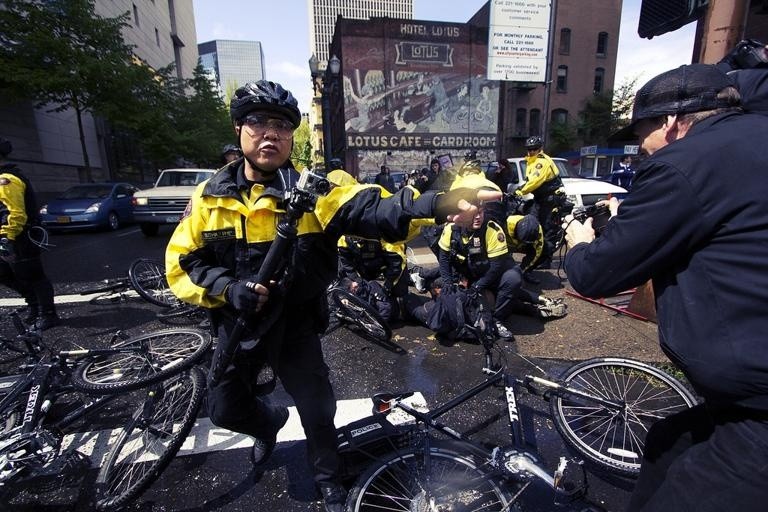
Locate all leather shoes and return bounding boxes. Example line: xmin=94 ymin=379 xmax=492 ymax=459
xmin=495 ymin=322 xmax=514 ymax=340
xmin=250 ymin=406 xmax=290 ymax=464
xmin=318 ymin=485 xmax=347 ymax=512
xmin=35 ymin=318 xmax=60 ymax=330
xmin=536 ymin=262 xmax=551 ymax=269
xmin=524 ymin=272 xmax=541 ymax=283
xmin=24 ymin=306 xmax=37 ymax=323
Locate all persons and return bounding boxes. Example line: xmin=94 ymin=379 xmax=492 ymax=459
xmin=609 ymin=154 xmax=636 ymax=201
xmin=330 ymin=134 xmax=567 ymax=342
xmin=563 ymin=62 xmax=767 ymax=512
xmin=163 ymin=79 xmax=504 ymax=512
xmin=0 ymin=137 xmax=60 ymax=330
xmin=220 ymin=143 xmax=241 ymax=165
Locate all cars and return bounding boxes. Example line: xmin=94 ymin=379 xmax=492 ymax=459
xmin=502 ymin=154 xmax=630 ymax=223
xmin=39 ymin=181 xmax=140 ymax=230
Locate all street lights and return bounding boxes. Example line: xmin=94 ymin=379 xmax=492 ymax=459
xmin=308 ymin=53 xmax=343 ymax=174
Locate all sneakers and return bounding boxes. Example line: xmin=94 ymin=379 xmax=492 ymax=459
xmin=408 ymin=272 xmax=426 ymax=293
xmin=545 ymin=296 xmax=563 ymax=308
xmin=539 ymin=305 xmax=567 ymax=319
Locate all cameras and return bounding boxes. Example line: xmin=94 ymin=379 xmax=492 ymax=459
xmin=572 ymin=202 xmax=613 ymax=235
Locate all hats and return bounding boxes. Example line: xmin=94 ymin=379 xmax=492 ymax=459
xmin=610 ymin=64 xmax=743 ymax=141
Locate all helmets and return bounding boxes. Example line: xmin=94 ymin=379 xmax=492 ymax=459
xmin=229 ymin=80 xmax=302 ymax=127
xmin=328 ymin=158 xmax=344 ymax=169
xmin=516 ymin=215 xmax=539 ymax=245
xmin=222 ymin=143 xmax=243 ymax=155
xmin=524 ymin=136 xmax=542 ymax=149
xmin=0 ymin=136 xmax=14 ymax=157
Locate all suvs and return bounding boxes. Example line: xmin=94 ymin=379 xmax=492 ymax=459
xmin=132 ymin=169 xmax=221 ymax=238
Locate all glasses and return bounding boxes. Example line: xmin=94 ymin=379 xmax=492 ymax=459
xmin=242 ymin=116 xmax=297 ymax=141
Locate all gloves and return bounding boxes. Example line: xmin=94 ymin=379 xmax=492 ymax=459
xmin=464 ymin=288 xmax=478 ymax=308
xmin=441 ymin=280 xmax=455 ymax=296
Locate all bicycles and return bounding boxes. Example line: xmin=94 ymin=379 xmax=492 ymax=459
xmin=328 ymin=263 xmax=393 ymax=342
xmin=330 ymin=313 xmax=705 ymax=512
